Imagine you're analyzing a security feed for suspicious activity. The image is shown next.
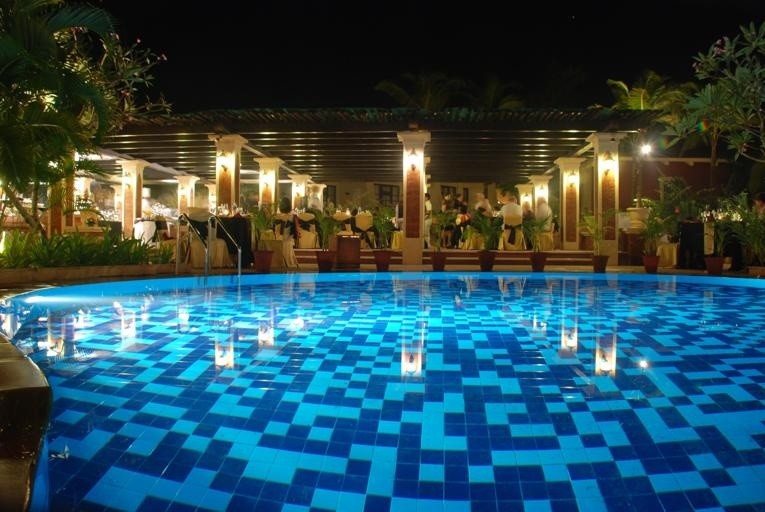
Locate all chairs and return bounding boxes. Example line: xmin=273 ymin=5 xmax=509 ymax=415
xmin=424 ymin=201 xmax=555 ymax=254
xmin=133 ymin=207 xmax=404 ymax=266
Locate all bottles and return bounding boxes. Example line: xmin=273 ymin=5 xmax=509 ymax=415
xmin=698 ymin=209 xmax=742 ymax=223
xmin=149 ymin=201 xmax=171 ymax=218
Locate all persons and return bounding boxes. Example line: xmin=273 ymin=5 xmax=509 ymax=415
xmin=753 ymin=193 xmax=765 ymax=223
xmin=420 ymin=190 xmax=561 ymax=251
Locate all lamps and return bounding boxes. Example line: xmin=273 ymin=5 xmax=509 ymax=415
xmin=172 ymin=292 xmax=194 ymax=334
xmin=111 ymin=296 xmax=153 ymax=352
xmin=47 ymin=299 xmax=93 ymax=361
xmin=401 ymin=339 xmax=424 ymax=378
xmin=216 ymin=148 xmax=237 ymax=175
xmin=404 ymin=149 xmax=421 ymax=172
xmin=215 ymin=318 xmax=239 ymax=372
xmin=259 ymin=169 xmax=279 ymax=195
xmin=567 ymin=170 xmax=579 ymax=188
xmin=125 ymin=170 xmax=141 ymax=188
xmin=559 ymin=325 xmax=578 ymax=354
xmin=177 ymin=185 xmax=216 ymax=203
xmin=521 ymin=184 xmax=548 ymax=202
xmin=599 ymin=151 xmax=617 ymax=173
xmin=292 ymin=181 xmax=324 ymax=204
xmin=595 ymin=327 xmax=617 ymax=375
xmin=255 ymin=303 xmax=304 ymax=348
xmin=532 ymin=309 xmax=551 ymax=333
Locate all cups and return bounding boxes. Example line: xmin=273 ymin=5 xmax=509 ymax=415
xmin=97 ymin=207 xmax=117 ymax=223
xmin=209 ymin=201 xmax=243 ymax=216
xmin=291 ymin=205 xmax=373 ymax=218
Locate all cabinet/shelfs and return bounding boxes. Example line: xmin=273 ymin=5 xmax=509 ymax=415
xmin=619 ymin=229 xmax=646 ymax=266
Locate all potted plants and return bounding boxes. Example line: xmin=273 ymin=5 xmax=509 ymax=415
xmin=636 ymin=208 xmax=682 ymax=275
xmin=729 ymin=206 xmax=765 ymax=277
xmin=364 ymin=205 xmax=403 ymax=271
xmin=303 ymin=205 xmax=339 ymax=273
xmin=462 ymin=208 xmax=507 ymax=270
xmin=516 ymin=211 xmax=553 ymax=273
xmin=251 ymin=207 xmax=274 ymax=275
xmin=703 ymin=210 xmax=732 ymax=275
xmin=577 ymin=206 xmax=617 ymax=273
xmin=428 ymin=209 xmax=459 ymax=272
